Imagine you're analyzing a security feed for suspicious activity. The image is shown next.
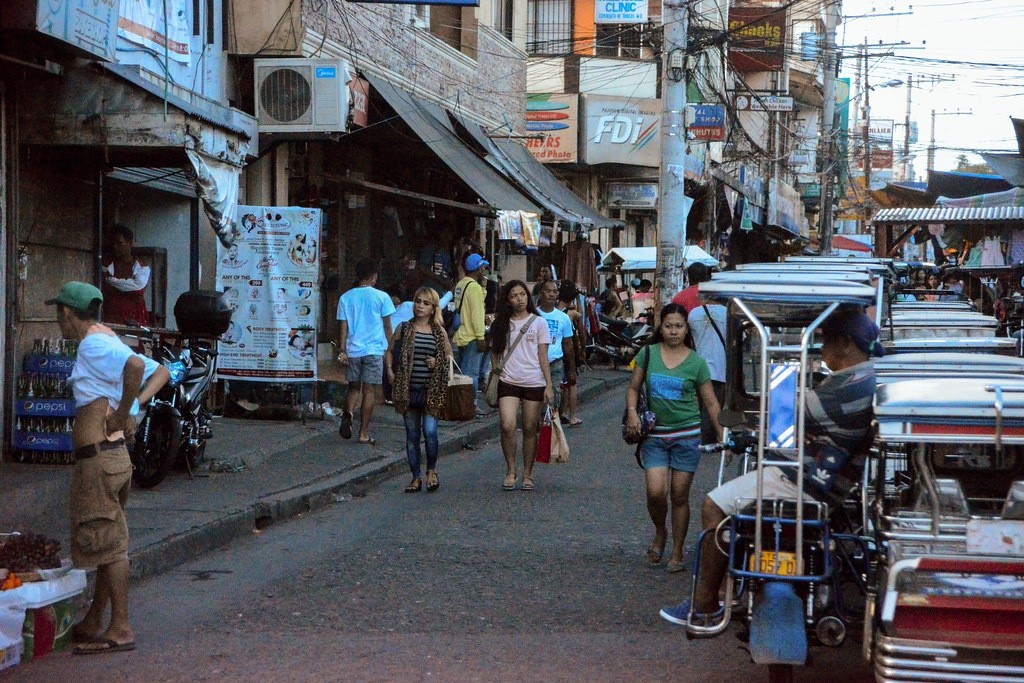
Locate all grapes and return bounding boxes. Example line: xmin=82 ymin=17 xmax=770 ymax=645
xmin=1 ymin=532 xmax=63 ymax=571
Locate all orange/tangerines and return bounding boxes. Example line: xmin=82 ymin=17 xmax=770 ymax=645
xmin=1 ymin=573 xmax=22 ymax=590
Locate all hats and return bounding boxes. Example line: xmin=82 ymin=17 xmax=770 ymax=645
xmin=46 ymin=281 xmax=101 ymax=313
xmin=465 ymin=254 xmax=490 ymax=272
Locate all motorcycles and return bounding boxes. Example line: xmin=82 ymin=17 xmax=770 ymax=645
xmin=699 ymin=255 xmax=1024 ymax=683
xmin=124 ymin=289 xmax=235 ymax=488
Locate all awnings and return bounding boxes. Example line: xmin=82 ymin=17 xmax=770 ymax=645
xmin=363 ymin=70 xmax=626 ymax=235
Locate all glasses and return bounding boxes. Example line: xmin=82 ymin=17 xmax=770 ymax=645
xmin=541 ymin=288 xmax=559 ymax=296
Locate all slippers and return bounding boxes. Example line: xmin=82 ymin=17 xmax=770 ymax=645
xmin=405 ymin=471 xmax=536 ymax=493
xmin=560 ymin=416 xmax=570 ymax=424
xmin=568 ymin=419 xmax=584 ymax=428
xmin=72 ymin=636 xmax=138 ymax=654
xmin=339 ymin=411 xmax=352 ymax=439
xmin=356 ymin=437 xmax=377 ymax=445
xmin=647 ymin=532 xmax=742 ymax=628
xmin=72 ymin=626 xmax=102 ymax=641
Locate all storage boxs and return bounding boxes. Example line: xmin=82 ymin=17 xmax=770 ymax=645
xmin=21 ymin=595 xmax=77 ymax=661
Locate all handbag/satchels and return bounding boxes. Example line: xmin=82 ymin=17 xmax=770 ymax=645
xmin=549 ymin=403 xmax=569 ymax=465
xmin=484 ymin=371 xmax=499 ymax=409
xmin=392 ymin=322 xmax=406 ymax=372
xmin=620 ymin=345 xmax=656 ymax=445
xmin=452 ymin=309 xmax=461 ymax=330
xmin=534 ymin=401 xmax=552 ymax=463
xmin=444 ymin=354 xmax=476 ymax=421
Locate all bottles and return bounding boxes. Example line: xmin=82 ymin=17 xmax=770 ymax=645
xmin=15 ymin=339 xmax=79 ymax=464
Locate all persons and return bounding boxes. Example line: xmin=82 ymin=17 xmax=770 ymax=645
xmin=888 ymin=253 xmax=1023 ymax=323
xmin=385 ymin=286 xmax=454 ymax=494
xmin=487 ymin=279 xmax=556 ymax=491
xmin=222 ymin=210 xmax=317 ymax=355
xmin=42 ymin=279 xmax=171 ymax=658
xmin=336 ymin=257 xmax=395 ymax=446
xmin=103 ymin=223 xmax=150 ymax=327
xmin=658 ymin=306 xmax=881 ymax=629
xmin=623 ymin=303 xmax=734 ymax=573
xmin=364 ymin=242 xmax=738 ymax=452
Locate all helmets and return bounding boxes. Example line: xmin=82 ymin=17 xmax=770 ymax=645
xmin=631 ymin=278 xmax=641 ymax=286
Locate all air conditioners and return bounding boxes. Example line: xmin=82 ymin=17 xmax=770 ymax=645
xmin=253 ymin=58 xmax=355 ymax=133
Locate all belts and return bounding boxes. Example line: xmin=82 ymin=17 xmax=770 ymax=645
xmin=75 ymin=438 xmax=125 ymax=459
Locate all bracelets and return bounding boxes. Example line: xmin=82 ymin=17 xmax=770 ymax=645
xmin=627 ymin=407 xmax=637 ymax=411
xmin=385 ymin=366 xmax=392 ymax=369
